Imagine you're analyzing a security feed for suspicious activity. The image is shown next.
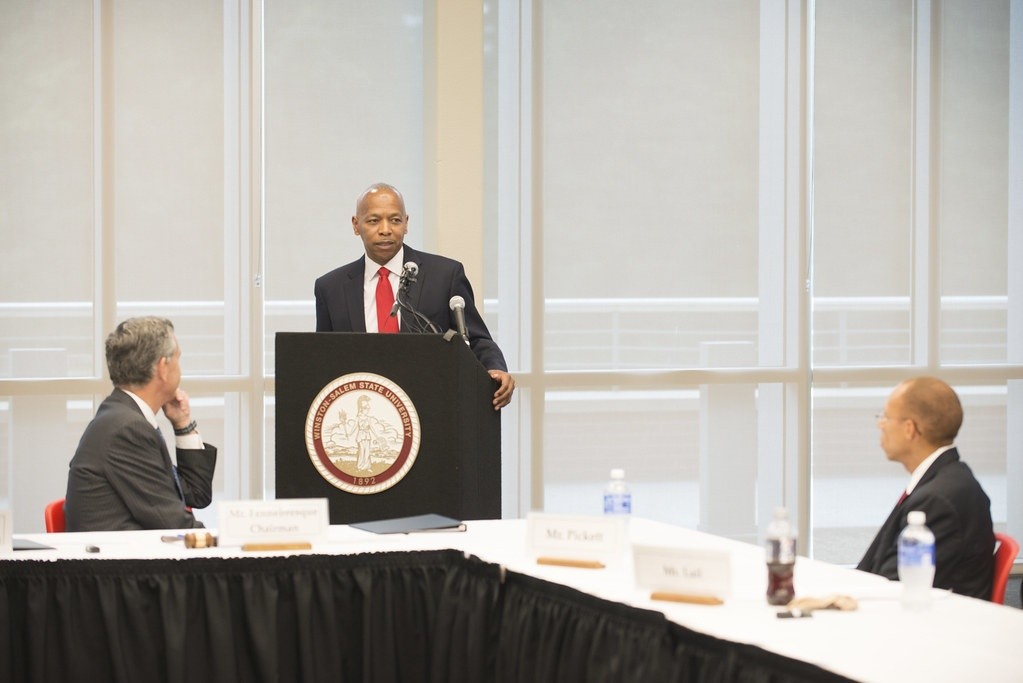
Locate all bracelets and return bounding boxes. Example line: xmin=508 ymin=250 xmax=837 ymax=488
xmin=175 ymin=420 xmax=197 ymax=435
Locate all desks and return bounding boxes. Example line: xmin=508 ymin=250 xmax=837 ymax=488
xmin=0 ymin=512 xmax=1023 ymax=683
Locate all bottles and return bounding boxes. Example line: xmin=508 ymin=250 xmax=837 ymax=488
xmin=896 ymin=510 xmax=935 ymax=616
xmin=765 ymin=509 xmax=797 ymax=607
xmin=602 ymin=467 xmax=632 ymax=552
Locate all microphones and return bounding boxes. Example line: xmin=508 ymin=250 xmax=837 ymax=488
xmin=402 ymin=261 xmax=418 ymax=277
xmin=448 ymin=296 xmax=470 ymax=347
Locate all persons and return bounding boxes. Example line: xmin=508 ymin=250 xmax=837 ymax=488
xmin=315 ymin=183 xmax=515 ymax=412
xmin=856 ymin=377 xmax=993 ymax=603
xmin=62 ymin=314 xmax=217 ymax=532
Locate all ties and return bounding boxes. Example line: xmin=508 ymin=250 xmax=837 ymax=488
xmin=895 ymin=491 xmax=909 ymax=512
xmin=375 ymin=268 xmax=400 ymax=335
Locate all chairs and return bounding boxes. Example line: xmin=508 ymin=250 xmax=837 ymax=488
xmin=45 ymin=499 xmax=65 ymax=533
xmin=990 ymin=533 xmax=1021 ymax=606
